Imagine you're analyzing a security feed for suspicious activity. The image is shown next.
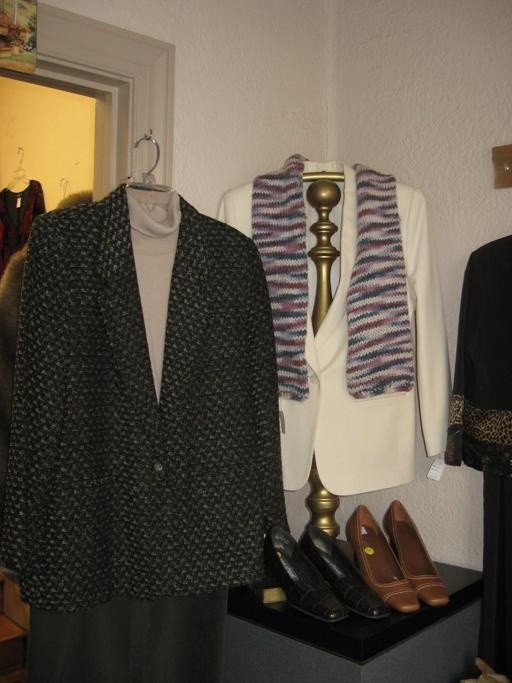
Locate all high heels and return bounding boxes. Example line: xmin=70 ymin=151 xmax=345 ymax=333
xmin=383 ymin=500 xmax=452 ymax=608
xmin=346 ymin=504 xmax=421 ymax=615
xmin=263 ymin=526 xmax=350 ymax=624
xmin=299 ymin=525 xmax=392 ymax=621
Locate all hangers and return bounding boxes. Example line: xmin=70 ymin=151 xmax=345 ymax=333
xmin=0 ymin=136 xmax=259 ymax=260
